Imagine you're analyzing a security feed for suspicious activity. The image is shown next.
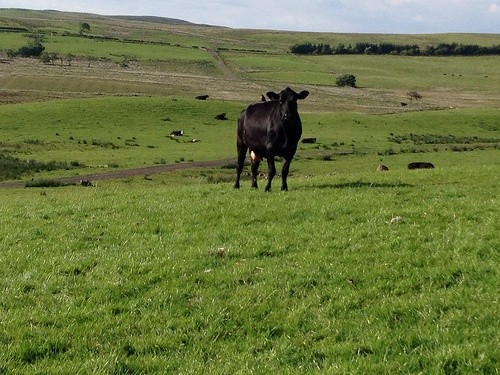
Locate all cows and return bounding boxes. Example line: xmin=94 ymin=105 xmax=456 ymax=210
xmin=216 ymin=113 xmax=226 ymax=119
xmin=302 ymin=138 xmax=317 ymax=144
xmin=169 ymin=130 xmax=184 ymax=136
xmin=195 ymin=95 xmax=209 ymax=100
xmin=233 ymin=87 xmax=309 ymax=193
